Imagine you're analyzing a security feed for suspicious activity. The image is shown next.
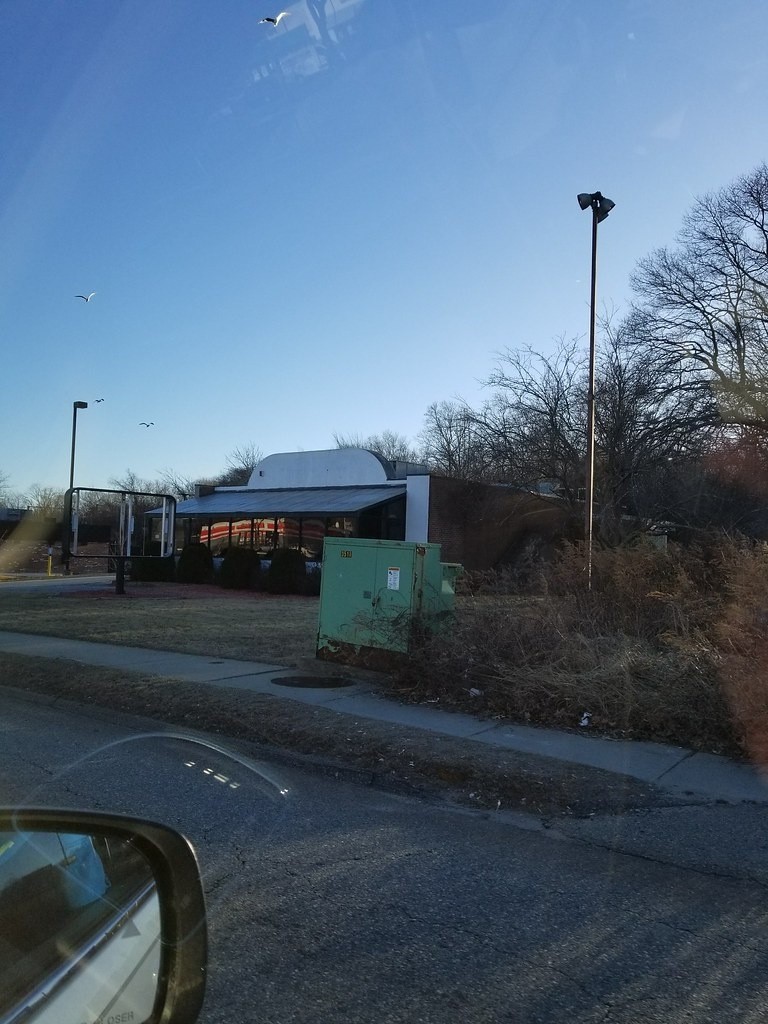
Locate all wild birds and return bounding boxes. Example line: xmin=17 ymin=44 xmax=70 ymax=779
xmin=139 ymin=423 xmax=154 ymax=427
xmin=74 ymin=293 xmax=96 ymax=302
xmin=94 ymin=398 xmax=104 ymax=403
xmin=257 ymin=12 xmax=290 ymax=27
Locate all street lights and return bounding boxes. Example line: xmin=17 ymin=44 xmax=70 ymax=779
xmin=575 ymin=191 xmax=615 ymax=589
xmin=69 ymin=401 xmax=89 ymax=487
